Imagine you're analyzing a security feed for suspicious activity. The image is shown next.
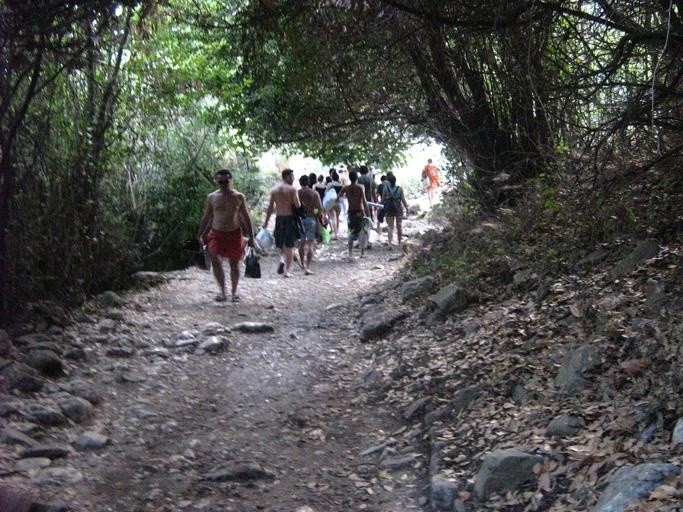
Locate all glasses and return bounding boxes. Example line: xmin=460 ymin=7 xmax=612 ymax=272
xmin=217 ymin=180 xmax=230 ymax=184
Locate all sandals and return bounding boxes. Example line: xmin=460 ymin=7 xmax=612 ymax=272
xmin=216 ymin=295 xmax=227 ymax=301
xmin=278 ymin=263 xmax=285 ymax=273
xmin=232 ymin=294 xmax=240 ymax=301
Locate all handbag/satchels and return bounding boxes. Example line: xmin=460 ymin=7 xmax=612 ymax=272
xmin=422 ymin=177 xmax=430 ymax=189
xmin=245 ymin=255 xmax=261 ymax=278
xmin=323 ymin=189 xmax=337 ymax=211
xmin=197 ymin=249 xmax=211 ymax=269
xmin=321 ymin=226 xmax=331 ymax=244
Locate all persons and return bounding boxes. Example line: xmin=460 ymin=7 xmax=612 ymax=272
xmin=298 ymin=166 xmax=410 ymax=261
xmin=197 ymin=169 xmax=257 ymax=302
xmin=421 ymin=158 xmax=443 ymax=208
xmin=262 ymin=169 xmax=302 ymax=277
